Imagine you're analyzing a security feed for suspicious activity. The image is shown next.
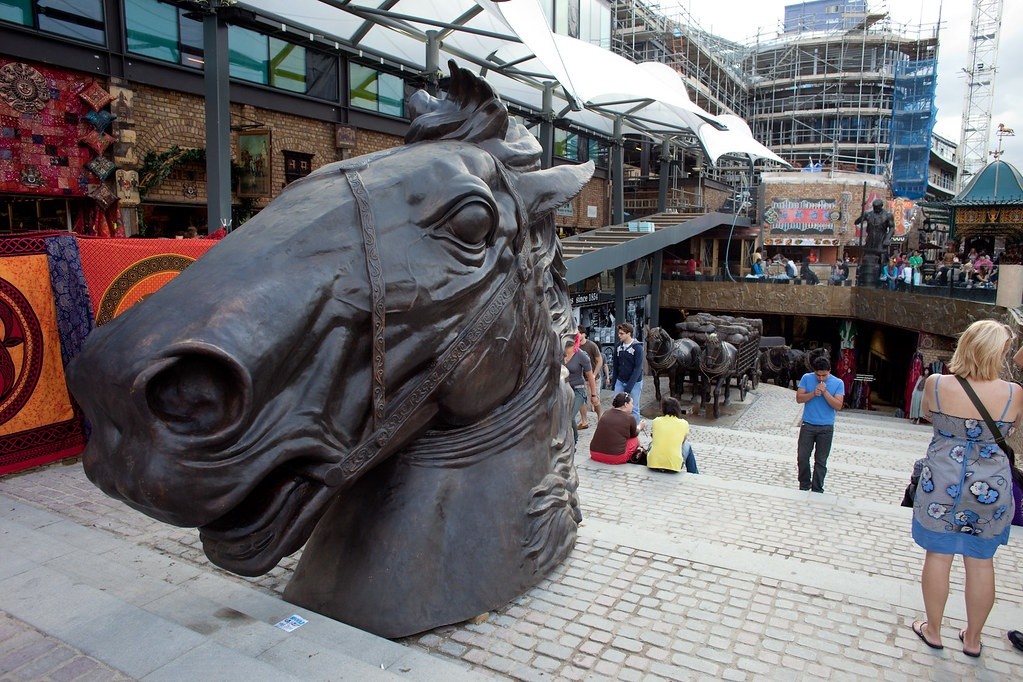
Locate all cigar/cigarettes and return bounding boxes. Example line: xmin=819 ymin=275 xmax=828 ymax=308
xmin=821 ymin=381 xmax=822 ymax=385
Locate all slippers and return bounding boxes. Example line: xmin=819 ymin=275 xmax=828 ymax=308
xmin=912 ymin=620 xmax=944 ymax=649
xmin=959 ymin=630 xmax=983 ymax=658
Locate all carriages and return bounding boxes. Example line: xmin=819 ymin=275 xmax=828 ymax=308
xmin=756 ymin=337 xmax=831 ymax=390
xmin=644 ymin=324 xmax=762 ymax=421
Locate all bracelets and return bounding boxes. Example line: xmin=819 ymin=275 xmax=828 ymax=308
xmin=592 ymin=395 xmax=597 ymax=397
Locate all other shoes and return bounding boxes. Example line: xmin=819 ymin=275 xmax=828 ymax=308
xmin=577 ymin=424 xmax=588 ymax=430
xmin=817 ymin=282 xmax=824 ymax=286
xmin=1007 ymin=629 xmax=1023 ymax=651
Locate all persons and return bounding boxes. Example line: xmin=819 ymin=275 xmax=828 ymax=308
xmin=796 ymin=357 xmax=845 ymax=494
xmin=750 ymin=246 xmax=798 ymax=278
xmin=883 ymin=249 xmax=1007 ymax=292
xmin=801 ymin=249 xmax=851 ymax=286
xmin=590 ymin=323 xmax=699 ymax=474
xmin=913 ymin=320 xmax=1023 ymax=658
xmin=904 ymin=351 xmax=948 ymax=424
xmin=560 ymin=325 xmax=610 ymax=453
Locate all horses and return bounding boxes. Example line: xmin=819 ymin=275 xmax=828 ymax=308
xmin=67 ymin=85 xmax=599 ymax=636
xmin=643 ymin=309 xmax=831 ymax=420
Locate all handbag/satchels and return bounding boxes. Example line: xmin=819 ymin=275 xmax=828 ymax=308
xmin=879 ymin=273 xmax=888 ymax=281
xmin=630 ymin=446 xmax=647 ymax=465
xmin=1008 ymin=466 xmax=1023 ymax=527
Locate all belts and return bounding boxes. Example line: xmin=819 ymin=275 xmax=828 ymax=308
xmin=574 ymin=384 xmax=585 ymax=389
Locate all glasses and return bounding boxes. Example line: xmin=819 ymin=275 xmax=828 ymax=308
xmin=617 ymin=332 xmax=627 ymax=335
xmin=625 ymin=392 xmax=630 ymax=402
xmin=1005 ymin=326 xmax=1014 ymax=353
